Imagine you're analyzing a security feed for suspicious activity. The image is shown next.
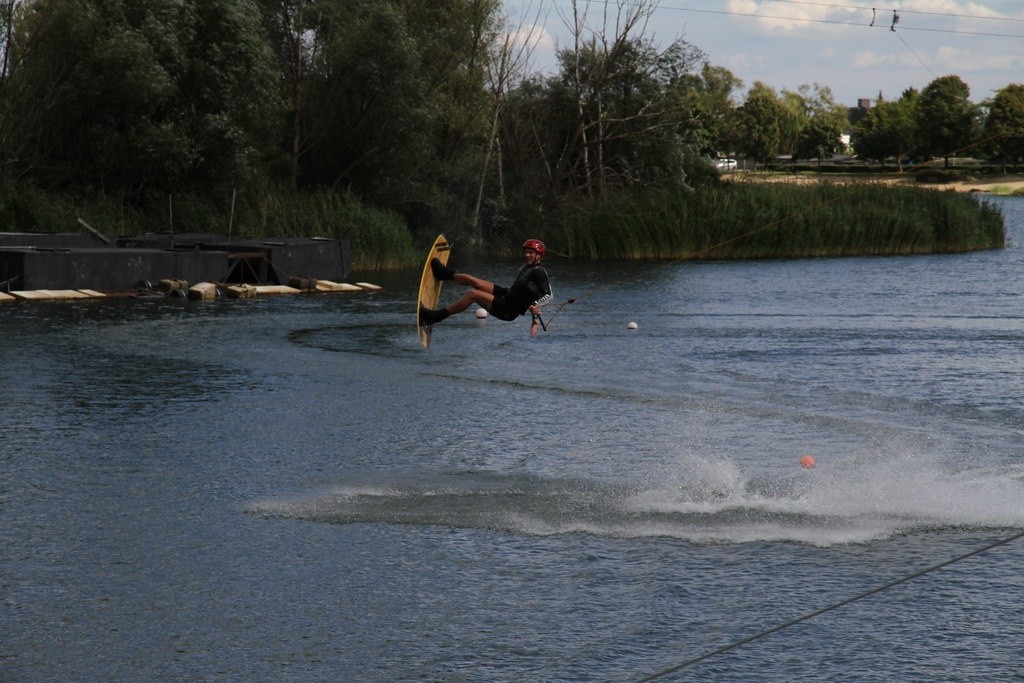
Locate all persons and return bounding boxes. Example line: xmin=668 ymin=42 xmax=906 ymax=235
xmin=419 ymin=239 xmax=553 ymax=337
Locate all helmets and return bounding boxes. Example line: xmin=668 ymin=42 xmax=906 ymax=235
xmin=522 ymin=239 xmax=545 ymax=259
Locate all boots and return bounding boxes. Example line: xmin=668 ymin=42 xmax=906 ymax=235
xmin=430 ymin=258 xmax=454 ymax=281
xmin=420 ymin=303 xmax=449 ymax=326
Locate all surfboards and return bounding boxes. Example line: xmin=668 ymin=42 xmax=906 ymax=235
xmin=416 ymin=233 xmax=451 ymax=350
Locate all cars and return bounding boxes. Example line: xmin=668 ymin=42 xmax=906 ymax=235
xmin=715 ymin=159 xmax=737 ymax=171
